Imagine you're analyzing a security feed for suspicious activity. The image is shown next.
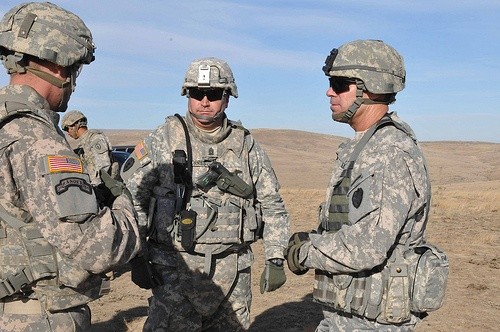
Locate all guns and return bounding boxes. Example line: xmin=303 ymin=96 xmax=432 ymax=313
xmin=195 ymin=162 xmax=252 ymax=198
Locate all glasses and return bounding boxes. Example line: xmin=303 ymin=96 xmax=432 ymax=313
xmin=188 ymin=87 xmax=224 ymax=101
xmin=328 ymin=77 xmax=356 ymax=93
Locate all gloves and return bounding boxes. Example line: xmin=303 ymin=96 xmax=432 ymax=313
xmin=284 ymin=240 xmax=309 ymax=276
xmin=288 ymin=232 xmax=309 ymax=247
xmin=100 ymin=162 xmax=134 ymax=206
xmin=260 ymin=259 xmax=286 ymax=294
xmin=132 ymin=255 xmax=162 ymax=289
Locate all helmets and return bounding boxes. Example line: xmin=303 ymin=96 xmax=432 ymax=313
xmin=61 ymin=111 xmax=87 ymax=131
xmin=0 ymin=2 xmax=96 ymax=68
xmin=323 ymin=40 xmax=406 ymax=94
xmin=181 ymin=56 xmax=238 ymax=98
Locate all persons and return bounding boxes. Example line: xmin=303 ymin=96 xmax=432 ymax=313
xmin=60 ymin=110 xmax=114 ymax=282
xmin=0 ymin=1 xmax=147 ymax=332
xmin=119 ymin=56 xmax=291 ymax=332
xmin=282 ymin=39 xmax=431 ymax=332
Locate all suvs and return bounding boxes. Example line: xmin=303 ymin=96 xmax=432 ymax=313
xmin=72 ymin=143 xmax=137 ymax=209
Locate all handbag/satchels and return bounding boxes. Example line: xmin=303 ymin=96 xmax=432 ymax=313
xmin=406 ymin=244 xmax=449 ymax=314
